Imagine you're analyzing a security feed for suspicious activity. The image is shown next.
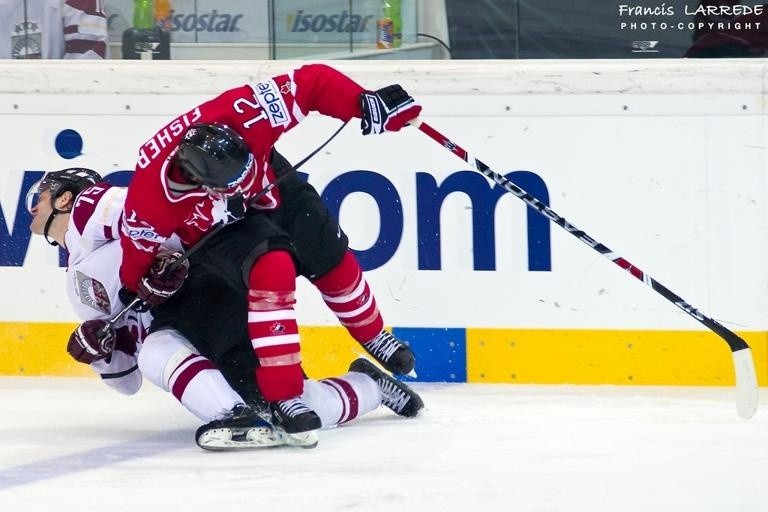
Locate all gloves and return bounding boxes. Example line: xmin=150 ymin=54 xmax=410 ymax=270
xmin=135 ymin=248 xmax=190 ymax=306
xmin=66 ymin=317 xmax=116 ymax=365
xmin=359 ymin=83 xmax=422 ymax=136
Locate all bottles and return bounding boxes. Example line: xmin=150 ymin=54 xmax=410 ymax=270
xmin=132 ymin=0 xmax=155 ymax=32
xmin=377 ymin=1 xmax=403 ymax=51
xmin=154 ymin=0 xmax=174 ymax=30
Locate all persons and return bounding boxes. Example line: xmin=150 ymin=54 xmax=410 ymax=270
xmin=0 ymin=0 xmax=110 ymax=59
xmin=117 ymin=65 xmax=422 ymax=434
xmin=27 ymin=167 xmax=425 ymax=452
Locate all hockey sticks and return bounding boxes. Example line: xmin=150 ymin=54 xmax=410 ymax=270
xmin=409 ymin=117 xmax=757 ymax=419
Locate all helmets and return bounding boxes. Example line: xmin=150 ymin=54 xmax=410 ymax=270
xmin=176 ymin=118 xmax=255 ymax=189
xmin=37 ymin=166 xmax=103 ymax=214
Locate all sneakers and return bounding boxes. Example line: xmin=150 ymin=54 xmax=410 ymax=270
xmin=360 ymin=327 xmax=416 ymax=376
xmin=195 ymin=403 xmax=273 ymax=451
xmin=348 ymin=358 xmax=424 ymax=420
xmin=269 ymin=399 xmax=323 ymax=435
